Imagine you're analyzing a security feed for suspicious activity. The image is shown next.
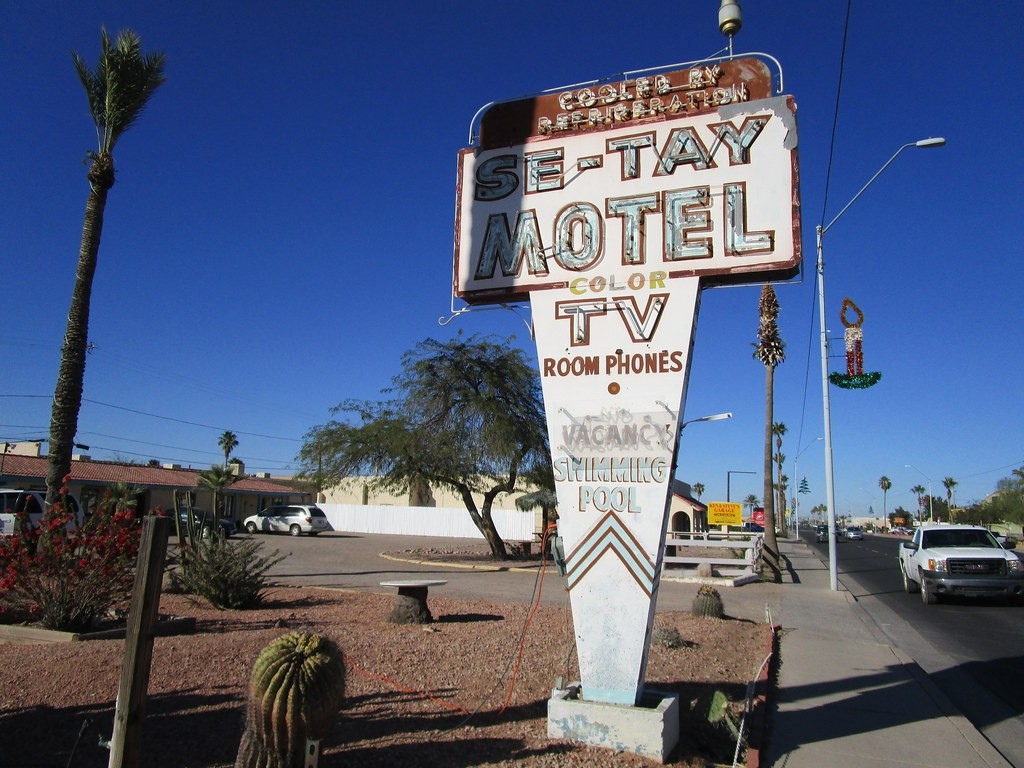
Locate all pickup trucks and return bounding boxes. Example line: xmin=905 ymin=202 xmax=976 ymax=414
xmin=898 ymin=522 xmax=1024 ymax=604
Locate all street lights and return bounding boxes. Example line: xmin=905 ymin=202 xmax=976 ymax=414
xmin=905 ymin=464 xmax=933 ymax=523
xmin=815 ymin=137 xmax=947 ymax=592
xmin=794 ymin=437 xmax=823 ymax=540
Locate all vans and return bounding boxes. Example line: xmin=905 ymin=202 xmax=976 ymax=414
xmin=0 ymin=488 xmax=85 ymax=539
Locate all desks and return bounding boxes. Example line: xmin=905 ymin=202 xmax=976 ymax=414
xmin=379 ymin=579 xmax=449 ymax=623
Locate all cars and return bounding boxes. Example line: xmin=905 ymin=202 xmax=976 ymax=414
xmin=139 ymin=507 xmax=237 ymax=539
xmin=242 ymin=504 xmax=328 ymax=536
xmin=817 ymin=525 xmax=864 ymax=542
